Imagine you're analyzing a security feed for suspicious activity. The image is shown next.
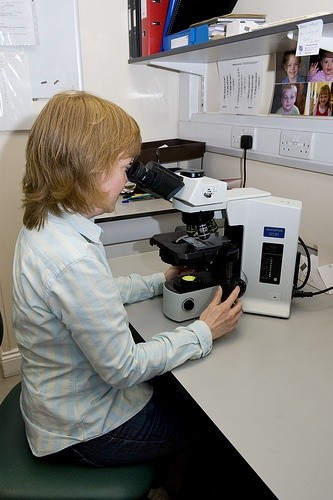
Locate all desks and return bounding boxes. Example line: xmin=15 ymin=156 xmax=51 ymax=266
xmin=108 ymin=250 xmax=333 ymax=500
xmin=92 ymin=193 xmax=224 ymax=260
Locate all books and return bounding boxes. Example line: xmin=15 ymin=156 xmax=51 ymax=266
xmin=191 ymin=18 xmax=266 ymax=41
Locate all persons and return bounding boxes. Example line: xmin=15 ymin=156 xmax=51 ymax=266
xmin=308 ymin=52 xmax=333 ymax=82
xmin=10 ymin=91 xmax=244 ymax=500
xmin=312 ymin=85 xmax=332 ymax=116
xmin=282 ymin=50 xmax=306 ymax=82
xmin=276 ymin=85 xmax=301 ymax=114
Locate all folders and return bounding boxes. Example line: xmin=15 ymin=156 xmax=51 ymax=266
xmin=141 ymin=0 xmax=169 ymax=57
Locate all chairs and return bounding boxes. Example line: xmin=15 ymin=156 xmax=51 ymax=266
xmin=0 ymin=312 xmax=153 ymax=500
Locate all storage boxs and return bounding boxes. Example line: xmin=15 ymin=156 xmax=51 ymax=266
xmin=160 ymin=0 xmax=238 ymax=53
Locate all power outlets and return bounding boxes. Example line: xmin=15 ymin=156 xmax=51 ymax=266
xmin=231 ymin=127 xmax=256 ymax=151
xmin=279 ymin=130 xmax=313 ymax=160
